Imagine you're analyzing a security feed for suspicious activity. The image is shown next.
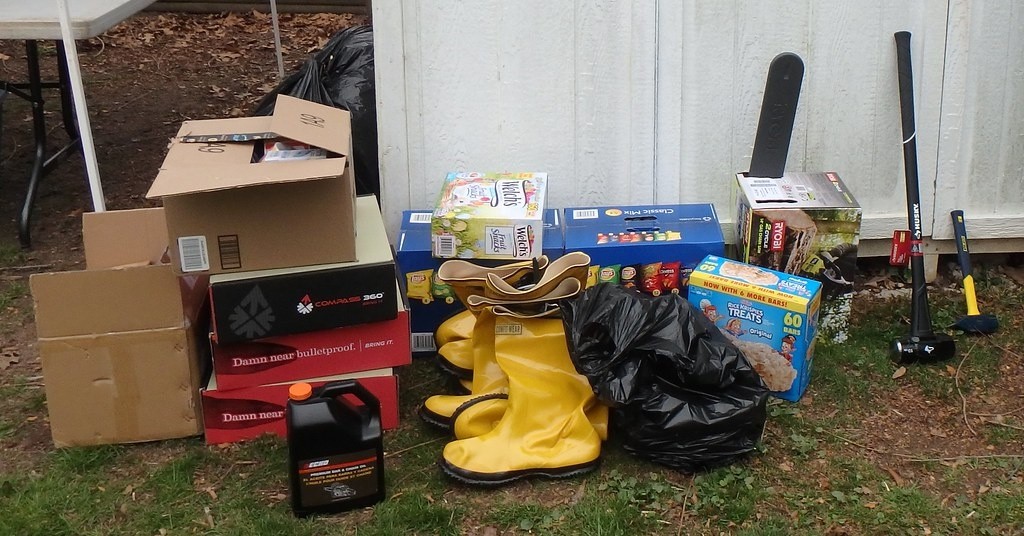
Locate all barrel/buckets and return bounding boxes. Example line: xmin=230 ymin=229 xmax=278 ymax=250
xmin=285 ymin=379 xmax=386 ymax=519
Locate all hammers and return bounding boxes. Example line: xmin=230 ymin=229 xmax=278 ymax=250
xmin=949 ymin=210 xmax=1002 ymax=337
xmin=890 ymin=30 xmax=955 ymax=369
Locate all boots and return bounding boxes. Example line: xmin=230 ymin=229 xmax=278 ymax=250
xmin=415 ymin=250 xmax=611 ymax=489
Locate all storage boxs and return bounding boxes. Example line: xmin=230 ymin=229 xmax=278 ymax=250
xmin=24 ymin=95 xmax=862 ymax=446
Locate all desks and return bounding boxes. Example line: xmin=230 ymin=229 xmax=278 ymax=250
xmin=0 ymin=1 xmax=162 ymax=248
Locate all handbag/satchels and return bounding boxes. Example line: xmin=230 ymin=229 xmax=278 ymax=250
xmin=557 ymin=281 xmax=771 ymax=477
xmin=247 ymin=23 xmax=381 ymax=197
